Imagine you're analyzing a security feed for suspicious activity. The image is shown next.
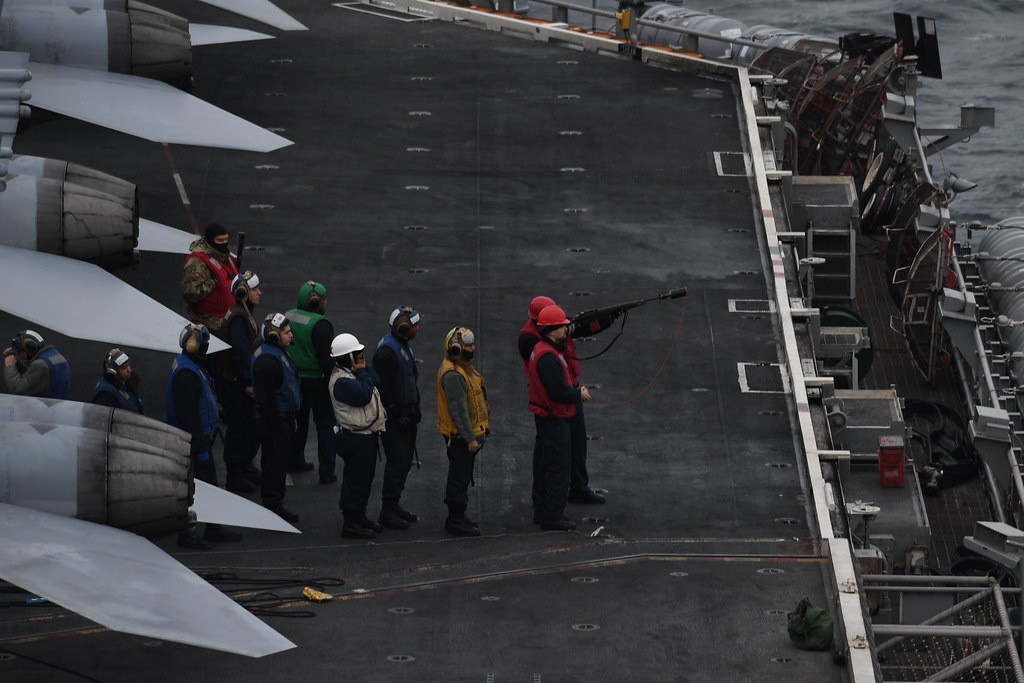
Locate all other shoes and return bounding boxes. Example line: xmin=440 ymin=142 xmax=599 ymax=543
xmin=242 ymin=463 xmax=263 ymax=484
xmin=225 ymin=473 xmax=256 ymax=493
xmin=177 ymin=531 xmax=211 ymax=549
xmin=288 ymin=462 xmax=315 ymax=474
xmin=319 ymin=472 xmax=609 ymax=538
xmin=204 ymin=525 xmax=241 ymax=542
xmin=278 ymin=508 xmax=299 ymax=523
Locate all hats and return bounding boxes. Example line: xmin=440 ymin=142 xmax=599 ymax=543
xmin=204 ymin=223 xmax=229 ymax=254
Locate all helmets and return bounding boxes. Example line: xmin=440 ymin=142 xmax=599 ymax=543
xmin=231 ymin=271 xmax=259 ymax=302
xmin=12 ymin=329 xmax=45 ymax=361
xmin=260 ymin=312 xmax=289 ymax=347
xmin=388 ymin=305 xmax=422 ymax=336
xmin=528 ymin=296 xmax=556 ymax=319
xmin=179 ymin=324 xmax=210 ymax=352
xmin=445 ymin=328 xmax=475 ymax=366
xmin=297 ymin=281 xmax=326 ymax=308
xmin=330 ymin=333 xmax=365 ymax=357
xmin=103 ymin=350 xmax=131 ymax=382
xmin=537 ymin=305 xmax=571 ymax=325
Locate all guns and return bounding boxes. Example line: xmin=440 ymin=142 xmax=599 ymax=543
xmin=566 ymin=286 xmax=688 ymax=327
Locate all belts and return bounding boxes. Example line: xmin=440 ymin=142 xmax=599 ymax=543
xmin=344 ymin=433 xmax=377 ymax=440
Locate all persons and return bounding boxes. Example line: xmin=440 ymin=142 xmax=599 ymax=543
xmin=329 ymin=333 xmax=383 ymax=540
xmin=182 ymin=223 xmax=239 ymax=393
xmin=437 ymin=326 xmax=491 ymax=537
xmin=284 ymin=280 xmax=338 ymax=484
xmin=517 ymin=296 xmax=613 ymax=504
xmin=164 ymin=322 xmax=242 ymax=549
xmin=214 ymin=269 xmax=263 ymax=493
xmin=527 ymin=305 xmax=591 ymax=531
xmin=93 ymin=348 xmax=143 ymax=415
xmin=372 ymin=305 xmax=424 ymax=529
xmin=245 ymin=312 xmax=299 ymax=523
xmin=3 ymin=329 xmax=69 ymax=401
